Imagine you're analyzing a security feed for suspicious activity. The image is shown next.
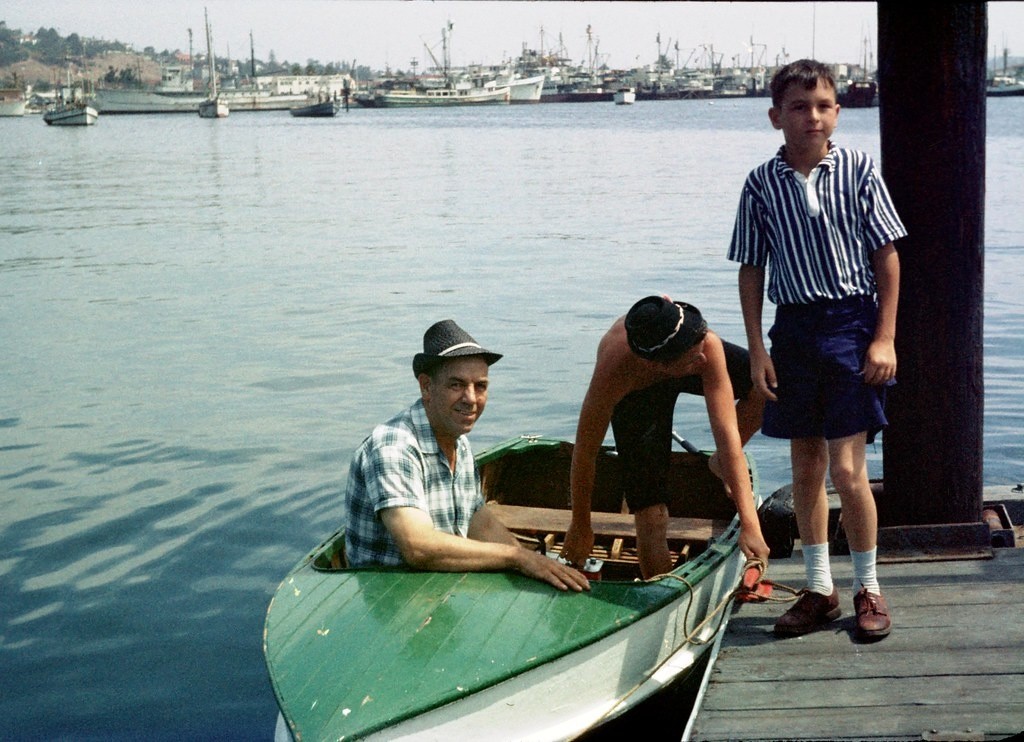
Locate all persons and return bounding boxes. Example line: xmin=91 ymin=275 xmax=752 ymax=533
xmin=560 ymin=297 xmax=770 ymax=582
xmin=346 ymin=320 xmax=592 ymax=590
xmin=727 ymin=59 xmax=908 ymax=637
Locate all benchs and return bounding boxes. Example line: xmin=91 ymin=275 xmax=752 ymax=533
xmin=485 ymin=500 xmax=713 ymax=540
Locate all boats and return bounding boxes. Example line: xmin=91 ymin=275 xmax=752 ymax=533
xmin=261 ymin=432 xmax=765 ymax=742
xmin=0 ymin=5 xmax=1024 ymax=118
xmin=43 ymin=49 xmax=99 ymax=126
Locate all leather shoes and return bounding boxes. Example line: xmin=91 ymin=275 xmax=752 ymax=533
xmin=852 ymin=580 xmax=892 ymax=639
xmin=773 ymin=586 xmax=843 ymax=637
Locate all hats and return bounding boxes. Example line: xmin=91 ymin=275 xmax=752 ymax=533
xmin=625 ymin=297 xmax=708 ymax=363
xmin=412 ymin=319 xmax=504 ymax=379
